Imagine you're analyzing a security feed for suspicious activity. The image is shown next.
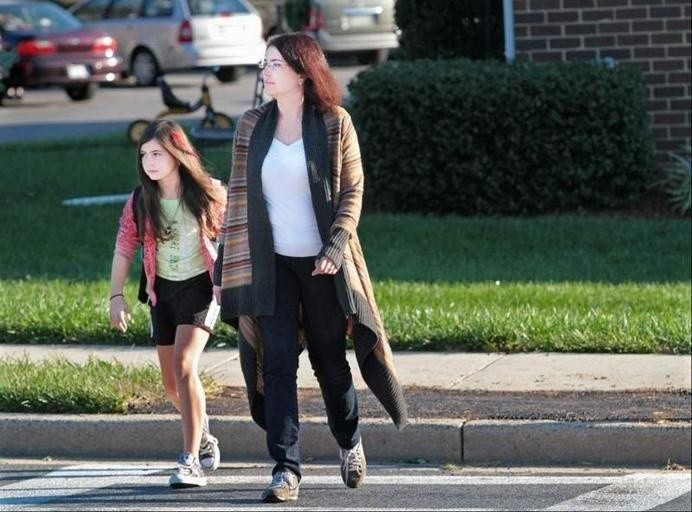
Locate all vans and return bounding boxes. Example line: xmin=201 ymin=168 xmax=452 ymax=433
xmin=69 ymin=1 xmax=266 ymax=87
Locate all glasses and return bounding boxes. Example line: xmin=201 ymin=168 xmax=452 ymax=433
xmin=257 ymin=59 xmax=289 ymax=69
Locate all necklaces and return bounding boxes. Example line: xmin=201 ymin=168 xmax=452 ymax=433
xmin=160 ymin=199 xmax=183 ymax=234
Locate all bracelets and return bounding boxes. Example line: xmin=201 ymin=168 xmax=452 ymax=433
xmin=108 ymin=291 xmax=126 ymax=305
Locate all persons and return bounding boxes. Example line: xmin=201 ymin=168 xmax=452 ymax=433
xmin=104 ymin=117 xmax=234 ymax=491
xmin=210 ymin=30 xmax=371 ymax=503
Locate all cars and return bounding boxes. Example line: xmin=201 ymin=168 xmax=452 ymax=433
xmin=0 ymin=1 xmax=120 ymax=104
xmin=253 ymin=1 xmax=403 ymax=66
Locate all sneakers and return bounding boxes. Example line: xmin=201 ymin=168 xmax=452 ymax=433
xmin=260 ymin=471 xmax=299 ymax=503
xmin=168 ymin=412 xmax=220 ymax=489
xmin=339 ymin=435 xmax=366 ymax=489
